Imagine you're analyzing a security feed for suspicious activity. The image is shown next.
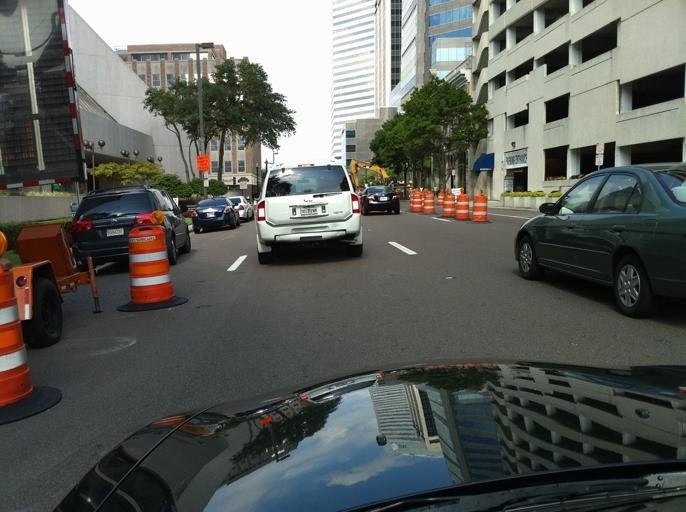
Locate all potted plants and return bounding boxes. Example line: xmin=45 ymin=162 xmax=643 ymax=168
xmin=501 ymin=189 xmax=562 ymax=212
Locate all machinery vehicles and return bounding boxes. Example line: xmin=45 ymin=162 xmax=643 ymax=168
xmin=0 ymin=224 xmax=89 ymax=347
xmin=350 ymin=159 xmax=404 ymax=195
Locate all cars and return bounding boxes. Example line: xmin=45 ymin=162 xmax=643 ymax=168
xmin=192 ymin=196 xmax=254 ymax=234
xmin=53 ymin=357 xmax=686 ymax=512
xmin=514 ymin=162 xmax=686 ymax=319
xmin=360 ymin=186 xmax=400 ymax=216
xmin=255 ymin=165 xmax=363 ymax=264
xmin=70 ymin=185 xmax=191 ymax=275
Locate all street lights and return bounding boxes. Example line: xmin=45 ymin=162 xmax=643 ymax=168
xmin=82 ymin=138 xmax=106 ymax=192
xmin=195 ymin=43 xmax=214 ymax=201
xmin=146 ymin=155 xmax=163 ymax=165
xmin=121 ymin=149 xmax=140 ymax=184
